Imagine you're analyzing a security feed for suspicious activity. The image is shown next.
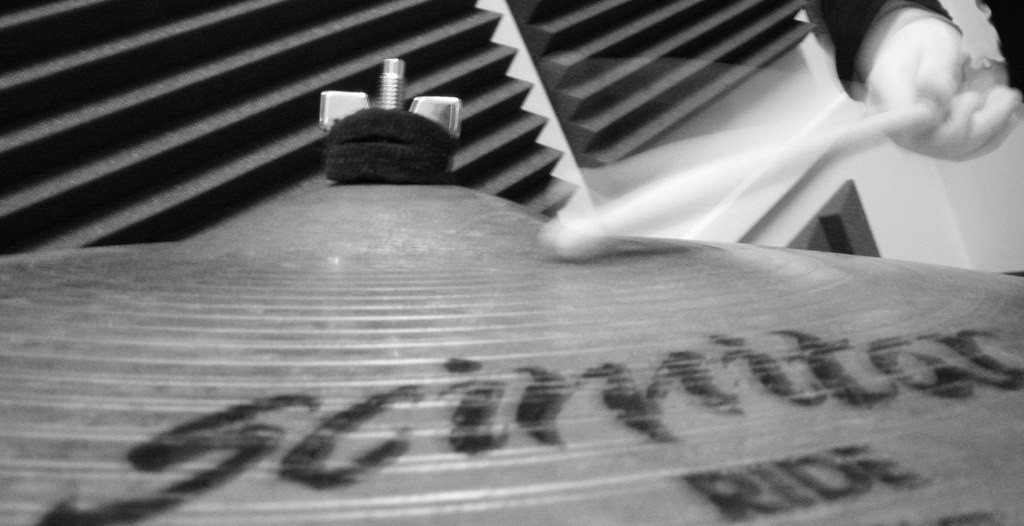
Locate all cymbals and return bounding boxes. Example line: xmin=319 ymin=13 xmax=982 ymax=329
xmin=0 ymin=185 xmax=1023 ymax=524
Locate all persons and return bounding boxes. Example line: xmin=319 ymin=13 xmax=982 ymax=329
xmin=805 ymin=0 xmax=1024 ymax=161
